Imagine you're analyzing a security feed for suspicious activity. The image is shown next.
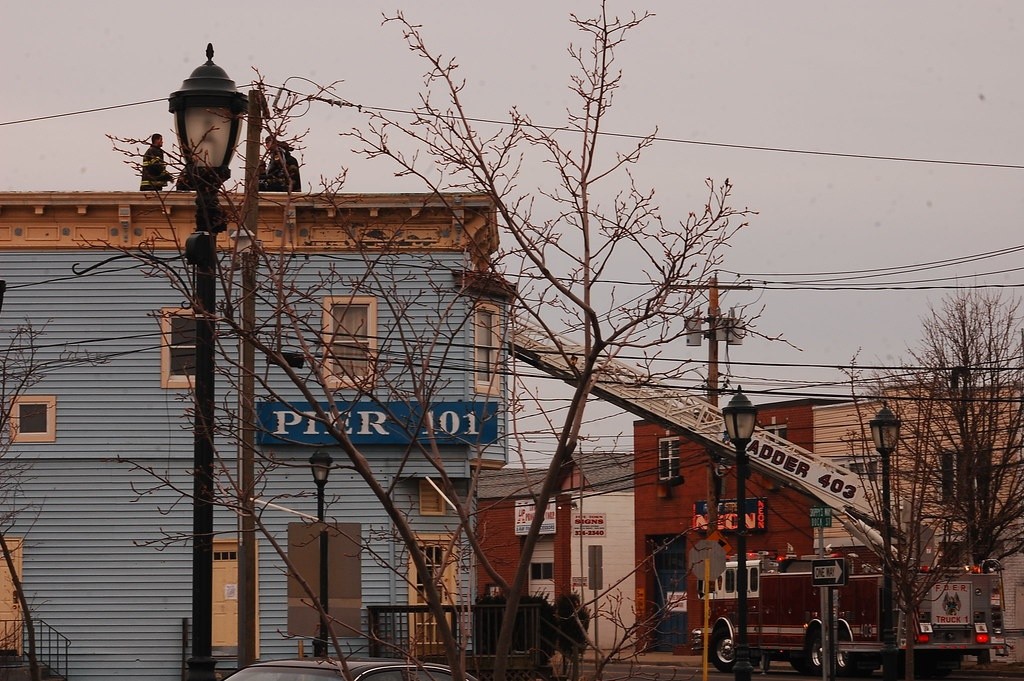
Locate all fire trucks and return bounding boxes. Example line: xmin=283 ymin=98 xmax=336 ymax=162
xmin=501 ymin=308 xmax=1015 ymax=677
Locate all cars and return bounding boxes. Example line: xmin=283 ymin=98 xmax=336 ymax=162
xmin=219 ymin=657 xmax=479 ymax=681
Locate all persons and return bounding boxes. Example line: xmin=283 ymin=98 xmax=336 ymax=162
xmin=140 ymin=133 xmax=174 ymax=191
xmin=256 ymin=135 xmax=302 ymax=192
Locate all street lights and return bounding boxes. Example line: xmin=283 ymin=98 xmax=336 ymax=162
xmin=722 ymin=383 xmax=758 ymax=681
xmin=165 ymin=42 xmax=249 ymax=681
xmin=868 ymin=399 xmax=902 ymax=680
xmin=308 ymin=443 xmax=334 ymax=659
